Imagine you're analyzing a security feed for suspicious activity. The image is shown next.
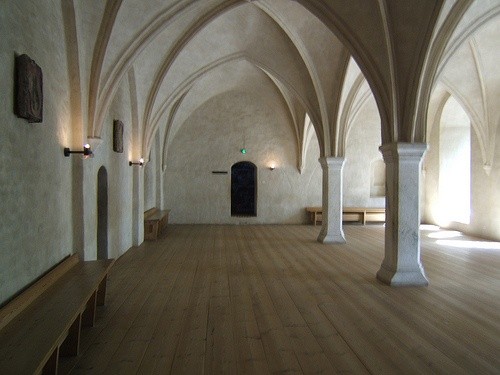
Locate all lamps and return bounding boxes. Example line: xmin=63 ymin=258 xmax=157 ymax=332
xmin=64 ymin=143 xmax=93 ymax=157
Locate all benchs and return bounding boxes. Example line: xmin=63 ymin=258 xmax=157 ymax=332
xmin=0 ymin=250 xmax=116 ymax=375
xmin=305 ymin=206 xmax=386 ymax=226
xmin=144 ymin=207 xmax=170 ymax=241
